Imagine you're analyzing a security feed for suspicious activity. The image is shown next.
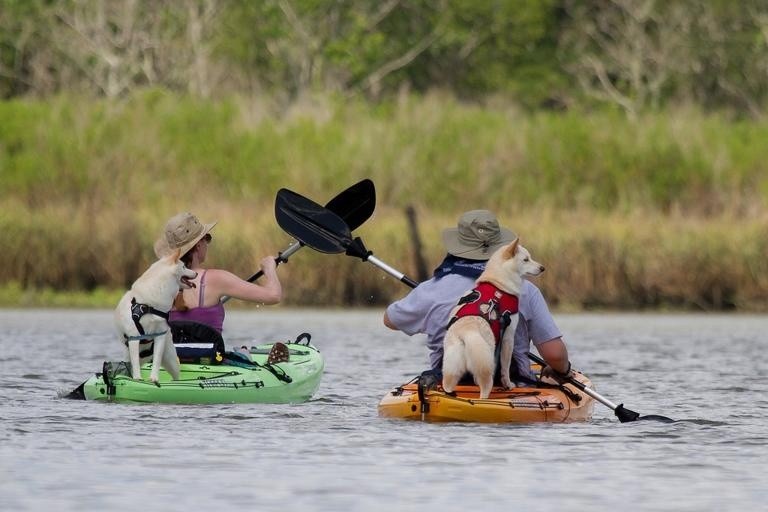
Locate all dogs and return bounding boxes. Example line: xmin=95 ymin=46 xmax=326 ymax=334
xmin=107 ymin=247 xmax=203 ymax=384
xmin=441 ymin=236 xmax=546 ymax=398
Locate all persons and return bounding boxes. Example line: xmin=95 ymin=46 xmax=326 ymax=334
xmin=383 ymin=209 xmax=573 ymax=390
xmin=151 ymin=211 xmax=285 ymax=361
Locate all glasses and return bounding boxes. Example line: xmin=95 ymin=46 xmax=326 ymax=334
xmin=200 ymin=233 xmax=212 ymax=244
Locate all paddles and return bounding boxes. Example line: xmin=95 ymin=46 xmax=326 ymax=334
xmin=65 ymin=179 xmax=375 ymax=400
xmin=275 ymin=187 xmax=677 ymax=423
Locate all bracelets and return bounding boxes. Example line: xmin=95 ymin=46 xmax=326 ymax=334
xmin=552 ymin=361 xmax=572 ymax=377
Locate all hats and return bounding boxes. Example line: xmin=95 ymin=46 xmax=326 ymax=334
xmin=153 ymin=212 xmax=220 ymax=260
xmin=443 ymin=209 xmax=518 ymax=261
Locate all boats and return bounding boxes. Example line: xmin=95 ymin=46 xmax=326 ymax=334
xmin=83 ymin=333 xmax=324 ymax=403
xmin=376 ymin=362 xmax=596 ymax=423
